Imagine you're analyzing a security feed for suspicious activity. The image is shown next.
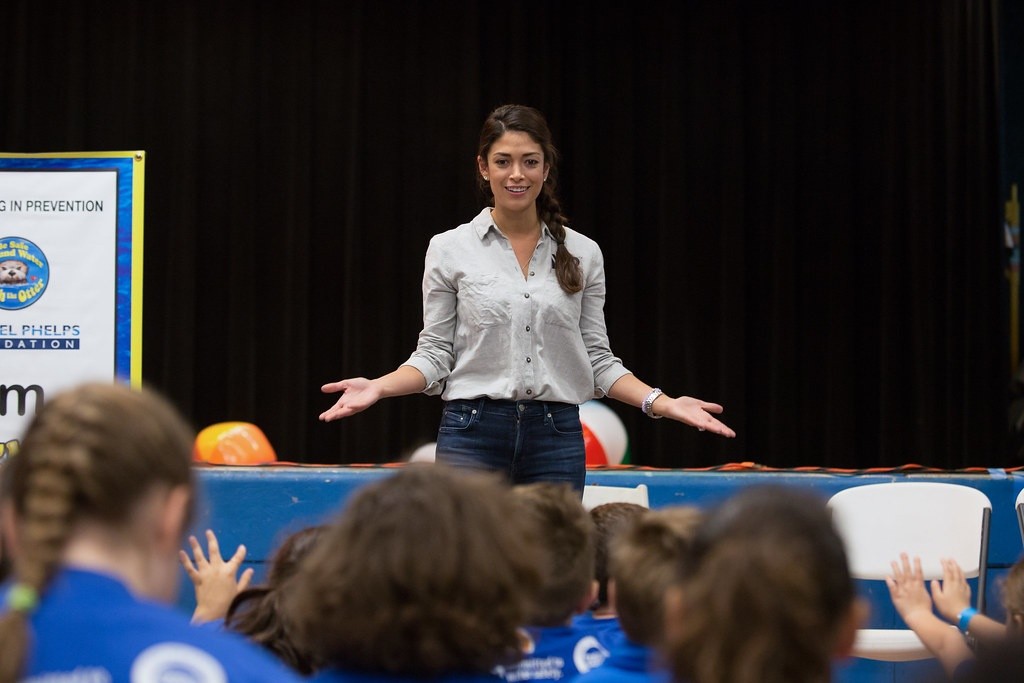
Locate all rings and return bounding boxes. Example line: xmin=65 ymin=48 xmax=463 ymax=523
xmin=698 ymin=427 xmax=705 ymax=431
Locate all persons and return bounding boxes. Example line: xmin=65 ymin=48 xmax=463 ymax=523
xmin=180 ymin=447 xmax=858 ymax=683
xmin=1 ymin=381 xmax=303 ymax=683
xmin=885 ymin=552 xmax=1024 ymax=683
xmin=318 ymin=104 xmax=735 ymax=507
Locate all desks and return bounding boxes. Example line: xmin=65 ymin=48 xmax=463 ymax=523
xmin=176 ymin=460 xmax=1023 ymax=682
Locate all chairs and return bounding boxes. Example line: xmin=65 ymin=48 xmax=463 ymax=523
xmin=823 ymin=482 xmax=992 ymax=662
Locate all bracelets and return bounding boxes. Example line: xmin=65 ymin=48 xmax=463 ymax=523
xmin=958 ymin=607 xmax=980 ymax=634
xmin=642 ymin=388 xmax=663 ymax=419
